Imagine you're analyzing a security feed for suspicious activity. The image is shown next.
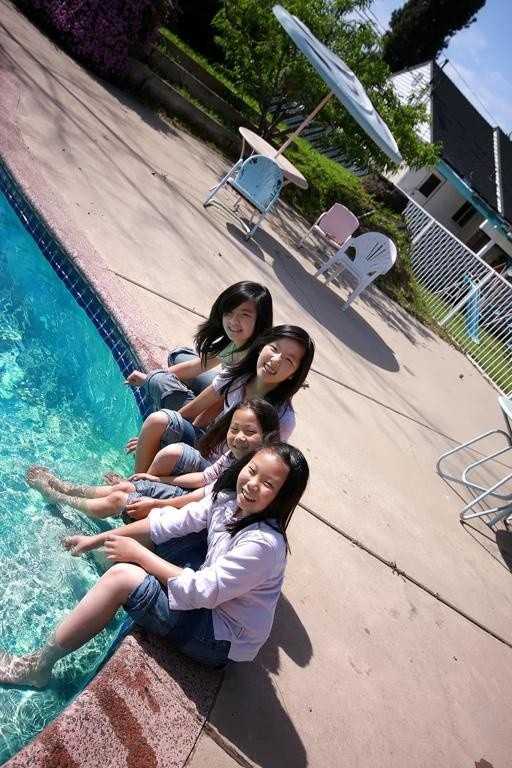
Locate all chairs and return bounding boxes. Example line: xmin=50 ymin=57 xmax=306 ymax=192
xmin=204 ymin=155 xmax=398 ymax=312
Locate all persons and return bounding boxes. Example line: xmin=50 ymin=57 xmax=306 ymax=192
xmin=21 ymin=397 xmax=279 ymax=524
xmin=104 ymin=322 xmax=316 ymax=486
xmin=0 ymin=440 xmax=312 ymax=690
xmin=121 ymin=277 xmax=274 ymax=413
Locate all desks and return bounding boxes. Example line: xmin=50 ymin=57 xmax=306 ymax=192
xmin=209 ymin=126 xmax=309 ymax=226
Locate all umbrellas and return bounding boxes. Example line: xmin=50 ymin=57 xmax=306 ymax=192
xmin=270 ymin=0 xmax=407 ymax=167
